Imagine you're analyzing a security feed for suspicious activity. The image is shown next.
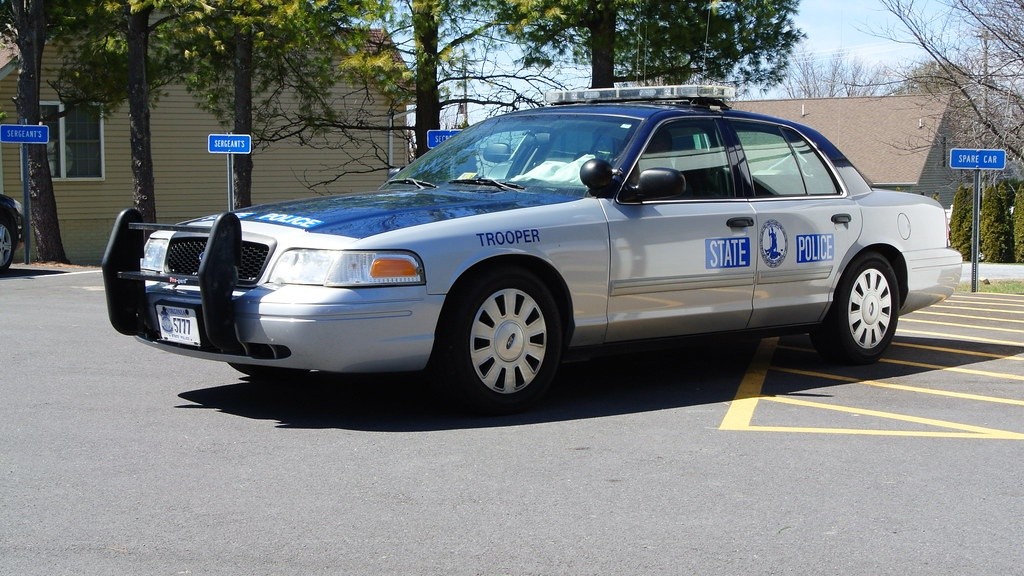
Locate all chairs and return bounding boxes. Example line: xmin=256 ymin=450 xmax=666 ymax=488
xmin=529 ymin=127 xmax=600 ymax=171
xmin=609 ymin=124 xmax=673 ymax=167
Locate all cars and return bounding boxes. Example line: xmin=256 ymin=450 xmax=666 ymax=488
xmin=99 ymin=84 xmax=964 ymax=416
